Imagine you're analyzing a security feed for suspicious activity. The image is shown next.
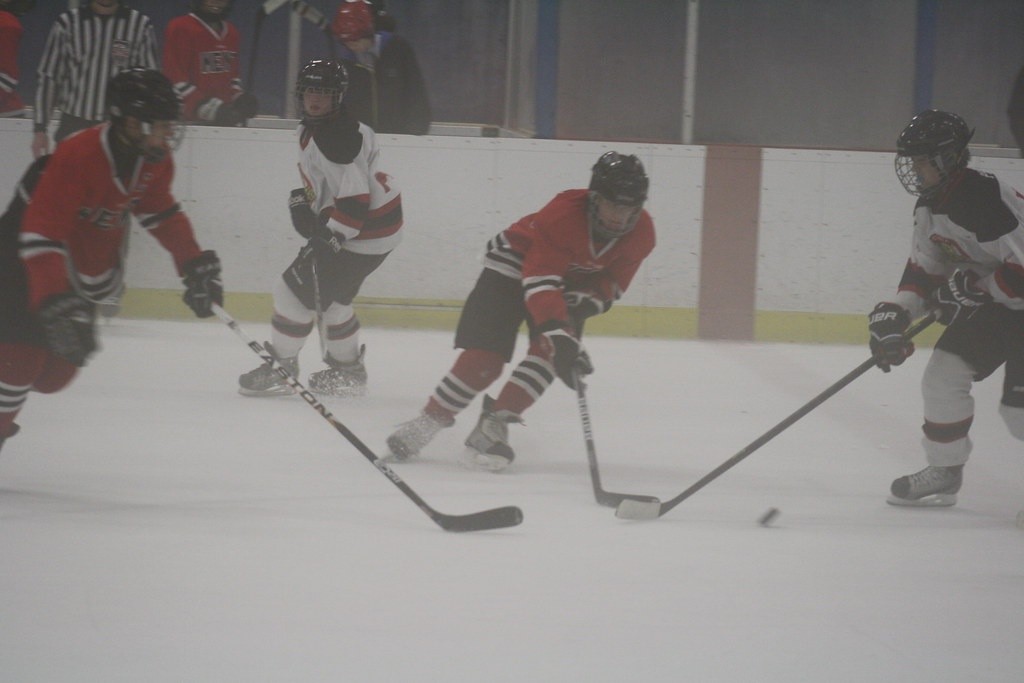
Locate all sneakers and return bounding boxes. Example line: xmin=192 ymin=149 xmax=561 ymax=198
xmin=237 ymin=340 xmax=300 ymax=393
xmin=887 ymin=466 xmax=964 ymax=507
xmin=309 ymin=344 xmax=370 ymax=397
xmin=464 ymin=393 xmax=526 ymax=473
xmin=382 ymin=407 xmax=456 ymax=464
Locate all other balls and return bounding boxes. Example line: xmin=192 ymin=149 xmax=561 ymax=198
xmin=762 ymin=510 xmax=777 ymax=523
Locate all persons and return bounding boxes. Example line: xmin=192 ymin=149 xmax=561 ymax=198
xmin=382 ymin=151 xmax=657 ymax=470
xmin=31 ymin=0 xmax=159 ymax=160
xmin=330 ymin=0 xmax=432 ymax=136
xmin=238 ymin=63 xmax=403 ymax=399
xmin=867 ymin=110 xmax=1024 ymax=532
xmin=1008 ymin=65 xmax=1024 ymax=154
xmin=162 ymin=0 xmax=257 ymax=126
xmin=0 ymin=0 xmax=36 ymax=118
xmin=0 ymin=68 xmax=223 ymax=449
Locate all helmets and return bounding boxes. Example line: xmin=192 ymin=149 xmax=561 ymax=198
xmin=331 ymin=0 xmax=376 ymax=47
xmin=893 ymin=110 xmax=976 ymax=203
xmin=294 ymin=57 xmax=349 ymax=123
xmin=106 ymin=66 xmax=184 ymax=158
xmin=585 ymin=151 xmax=651 ymax=238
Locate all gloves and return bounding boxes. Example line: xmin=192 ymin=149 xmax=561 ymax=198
xmin=196 ymin=97 xmax=242 ymax=127
xmin=296 ymin=228 xmax=347 ymax=282
xmin=34 ymin=294 xmax=96 ymax=368
xmin=867 ymin=300 xmax=914 ymax=373
xmin=562 ymin=273 xmax=616 ymax=319
xmin=229 ymin=92 xmax=259 ymax=118
xmin=180 ymin=250 xmax=224 ymax=317
xmin=539 ymin=326 xmax=595 ymax=388
xmin=288 ymin=188 xmax=321 ymax=239
xmin=923 ymin=270 xmax=992 ymax=329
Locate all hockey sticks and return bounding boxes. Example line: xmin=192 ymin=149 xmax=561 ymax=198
xmin=615 ymin=305 xmax=944 ymax=520
xmin=246 ymin=0 xmax=289 ymax=94
xmin=569 ymin=361 xmax=660 ymax=509
xmin=209 ymin=297 xmax=524 ymax=531
xmin=312 ymin=230 xmax=366 ymax=370
xmin=291 ymin=0 xmax=335 ymax=57
